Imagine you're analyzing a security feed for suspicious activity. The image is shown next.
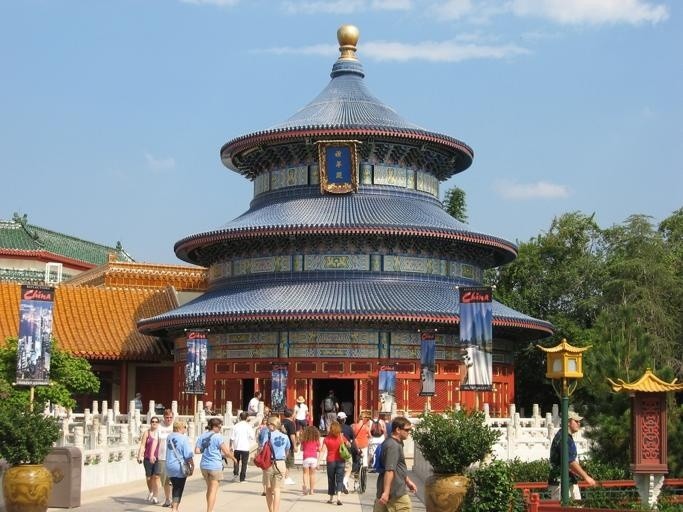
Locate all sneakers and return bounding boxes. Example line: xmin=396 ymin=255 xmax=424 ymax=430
xmin=342 ymin=483 xmax=349 ymax=494
xmin=163 ymin=501 xmax=170 ymax=508
xmin=145 ymin=492 xmax=160 ymax=504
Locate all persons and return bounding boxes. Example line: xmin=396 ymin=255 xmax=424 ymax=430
xmin=548 ymin=410 xmax=596 ymax=507
xmin=130 ymin=390 xmax=417 ymax=512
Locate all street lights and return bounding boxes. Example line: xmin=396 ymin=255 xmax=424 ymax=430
xmin=535 ymin=339 xmax=593 ymax=505
xmin=606 ymin=368 xmax=682 ymax=512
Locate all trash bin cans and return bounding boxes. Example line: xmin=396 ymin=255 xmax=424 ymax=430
xmin=41 ymin=446 xmax=82 ymax=509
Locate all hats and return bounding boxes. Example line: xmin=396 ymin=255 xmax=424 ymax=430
xmin=568 ymin=411 xmax=584 ymax=422
xmin=296 ymin=396 xmax=305 ymax=404
xmin=336 ymin=412 xmax=348 ymax=419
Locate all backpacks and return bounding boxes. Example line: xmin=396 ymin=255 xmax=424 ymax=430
xmin=254 ymin=431 xmax=282 ymax=474
xmin=369 ymin=418 xmax=384 ymax=438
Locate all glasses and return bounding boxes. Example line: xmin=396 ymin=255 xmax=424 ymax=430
xmin=400 ymin=428 xmax=411 ymax=432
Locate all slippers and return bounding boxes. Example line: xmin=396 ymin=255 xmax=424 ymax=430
xmin=327 ymin=500 xmax=343 ymax=505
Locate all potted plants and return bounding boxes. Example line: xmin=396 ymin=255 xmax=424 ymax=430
xmin=0 ymin=384 xmax=59 ymax=512
xmin=406 ymin=406 xmax=501 ymax=512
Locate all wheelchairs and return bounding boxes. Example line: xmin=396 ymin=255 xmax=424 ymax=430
xmin=334 ymin=449 xmax=368 ymax=495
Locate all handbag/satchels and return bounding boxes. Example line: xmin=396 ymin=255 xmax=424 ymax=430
xmin=338 ymin=433 xmax=352 ymax=459
xmin=179 ymin=462 xmax=193 ymax=476
